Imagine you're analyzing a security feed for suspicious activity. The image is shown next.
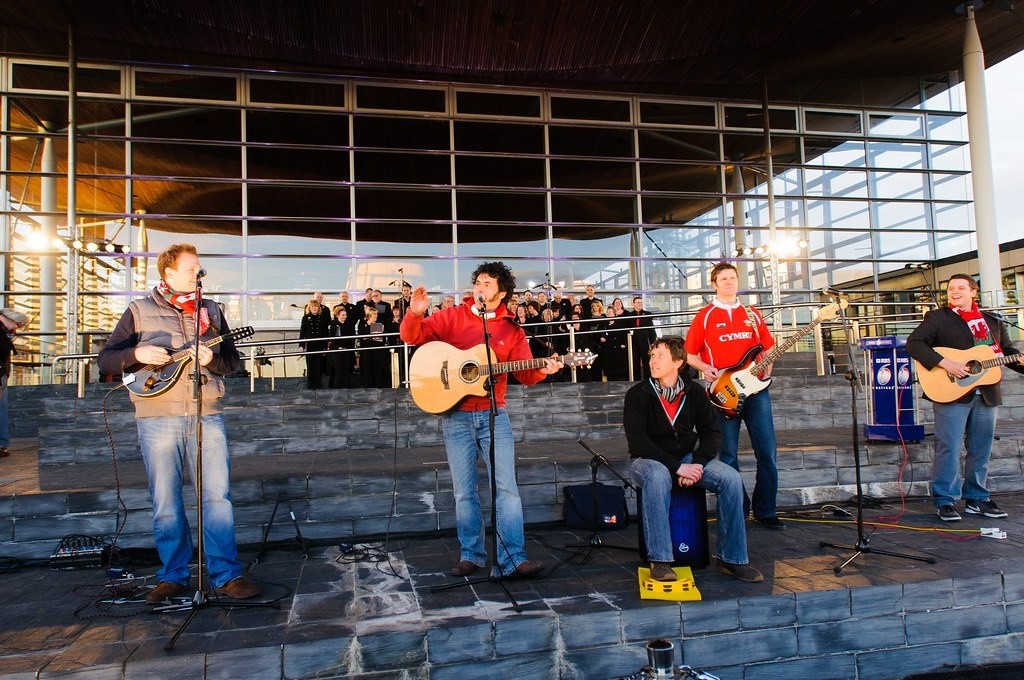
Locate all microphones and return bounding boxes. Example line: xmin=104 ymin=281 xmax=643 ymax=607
xmin=823 ymin=286 xmax=849 ymax=295
xmin=905 ymin=264 xmax=930 ymax=269
xmin=476 ymin=294 xmax=486 ymax=309
xmin=196 ymin=268 xmax=208 ymax=279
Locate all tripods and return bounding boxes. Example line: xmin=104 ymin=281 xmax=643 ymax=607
xmin=566 ymin=437 xmax=637 ymax=564
xmin=147 ymin=276 xmax=281 ymax=651
xmin=427 ymin=306 xmax=545 ymax=614
xmin=819 ymin=295 xmax=936 ymax=573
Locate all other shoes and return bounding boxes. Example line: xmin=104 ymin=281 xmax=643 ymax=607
xmin=490 ymin=560 xmax=544 ymax=580
xmin=0 ymin=446 xmax=8 ymax=456
xmin=651 ymin=561 xmax=678 ymax=582
xmin=452 ymin=560 xmax=478 ymax=575
xmin=763 ymin=515 xmax=786 ymax=530
xmin=146 ymin=581 xmax=190 ymax=604
xmin=212 ymin=577 xmax=265 ymax=599
xmin=717 ymin=557 xmax=765 ymax=582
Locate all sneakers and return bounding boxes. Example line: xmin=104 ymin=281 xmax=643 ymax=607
xmin=937 ymin=505 xmax=962 ymax=522
xmin=965 ymin=497 xmax=1009 ymax=518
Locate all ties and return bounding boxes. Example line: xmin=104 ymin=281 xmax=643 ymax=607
xmin=636 ymin=311 xmax=640 ymax=327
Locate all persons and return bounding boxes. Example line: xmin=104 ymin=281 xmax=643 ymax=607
xmin=0 ymin=323 xmax=16 ymax=456
xmin=400 ymin=262 xmax=565 ymax=577
xmin=684 ymin=262 xmax=789 ymax=530
xmin=97 ymin=244 xmax=262 ymax=604
xmin=623 ymin=337 xmax=764 ymax=582
xmin=904 ymin=274 xmax=1024 ymax=520
xmin=298 ymin=283 xmax=473 ymax=389
xmin=506 ymin=285 xmax=657 ymax=384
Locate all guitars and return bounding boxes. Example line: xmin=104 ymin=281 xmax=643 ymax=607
xmin=915 ymin=343 xmax=1023 ymax=406
xmin=706 ymin=298 xmax=850 ymax=421
xmin=407 ymin=340 xmax=599 ymax=417
xmin=121 ymin=325 xmax=255 ymax=398
